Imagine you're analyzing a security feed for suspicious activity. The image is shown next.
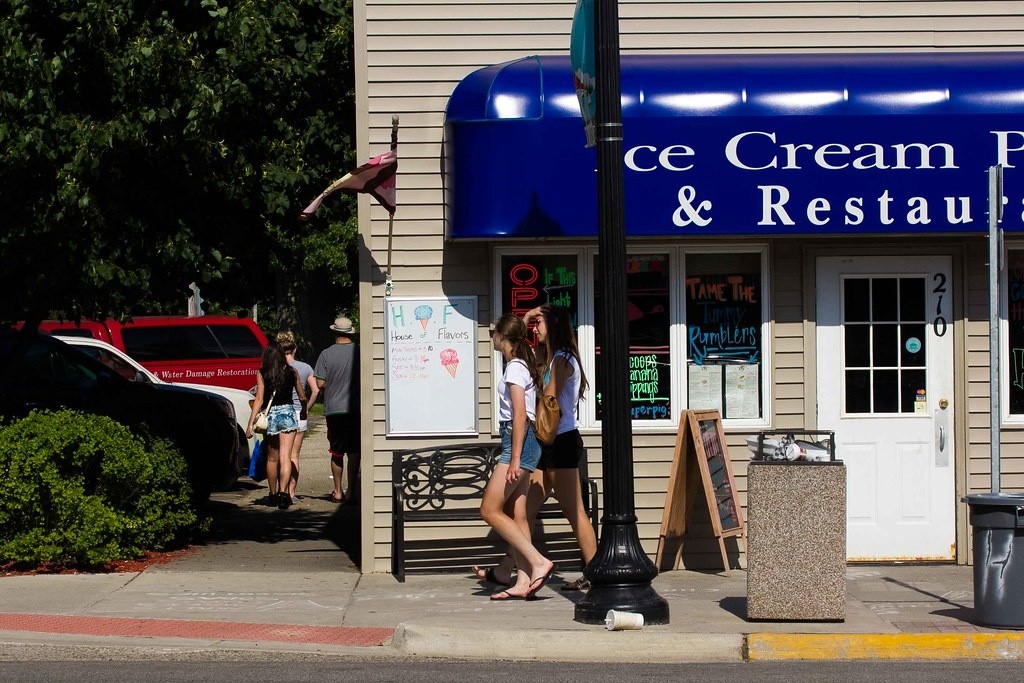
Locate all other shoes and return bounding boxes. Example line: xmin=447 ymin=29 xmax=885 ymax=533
xmin=267 ymin=491 xmax=278 ymax=507
xmin=292 ymin=495 xmax=304 ymax=504
xmin=277 ymin=491 xmax=291 ymax=509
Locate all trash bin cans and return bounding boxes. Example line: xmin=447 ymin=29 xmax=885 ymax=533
xmin=746 ymin=430 xmax=846 ymax=621
xmin=960 ymin=492 xmax=1024 ymax=630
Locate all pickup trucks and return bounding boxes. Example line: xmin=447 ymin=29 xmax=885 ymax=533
xmin=12 ymin=316 xmax=270 ymax=394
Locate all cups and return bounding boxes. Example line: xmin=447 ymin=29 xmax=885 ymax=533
xmin=606 ymin=609 xmax=644 ymax=630
xmin=785 ymin=443 xmax=814 ymax=462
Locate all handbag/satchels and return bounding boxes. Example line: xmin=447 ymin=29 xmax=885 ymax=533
xmin=249 ymin=433 xmax=267 ymax=482
xmin=252 ymin=412 xmax=269 ymax=435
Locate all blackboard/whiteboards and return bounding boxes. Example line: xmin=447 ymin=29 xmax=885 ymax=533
xmin=659 ymin=408 xmax=745 ymax=537
xmin=386 ymin=294 xmax=480 ymax=436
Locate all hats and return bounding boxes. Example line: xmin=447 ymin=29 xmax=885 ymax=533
xmin=328 ymin=318 xmax=356 ymax=335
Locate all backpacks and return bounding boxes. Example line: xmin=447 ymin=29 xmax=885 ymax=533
xmin=507 ymin=361 xmax=560 ymax=446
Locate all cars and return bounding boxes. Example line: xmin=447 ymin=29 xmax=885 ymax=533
xmin=0 ymin=325 xmax=257 ymax=510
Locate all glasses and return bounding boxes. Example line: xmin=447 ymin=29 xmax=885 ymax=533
xmin=489 ymin=329 xmax=495 ymax=338
xmin=535 ymin=318 xmax=545 ymax=326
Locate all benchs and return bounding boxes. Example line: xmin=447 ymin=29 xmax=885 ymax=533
xmin=390 ymin=442 xmax=598 ymax=584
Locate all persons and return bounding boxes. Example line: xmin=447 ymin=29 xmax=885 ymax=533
xmin=472 ymin=304 xmax=597 ymax=600
xmin=246 ymin=318 xmax=360 ymax=509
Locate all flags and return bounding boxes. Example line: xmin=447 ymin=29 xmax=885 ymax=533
xmin=300 ymin=134 xmax=397 ymax=219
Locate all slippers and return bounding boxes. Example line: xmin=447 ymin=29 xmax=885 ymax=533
xmin=489 ymin=589 xmax=538 ymax=600
xmin=560 ymin=577 xmax=593 ymax=591
xmin=478 ymin=567 xmax=510 ymax=587
xmin=330 ymin=490 xmax=345 ymax=503
xmin=524 ymin=563 xmax=559 ymax=600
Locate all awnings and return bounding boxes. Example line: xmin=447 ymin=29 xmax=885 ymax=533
xmin=446 ymin=53 xmax=1024 ymax=239
